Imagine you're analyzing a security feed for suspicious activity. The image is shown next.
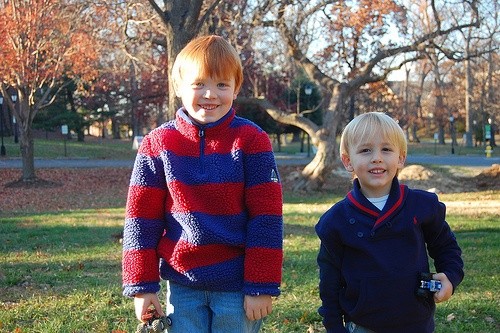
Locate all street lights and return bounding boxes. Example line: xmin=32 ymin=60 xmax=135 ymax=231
xmin=0 ymin=91 xmax=7 ymax=157
xmin=448 ymin=113 xmax=455 ymax=154
xmin=304 ymin=83 xmax=314 ymax=158
xmin=10 ymin=90 xmax=18 ymax=144
xmin=487 ymin=114 xmax=494 ymax=151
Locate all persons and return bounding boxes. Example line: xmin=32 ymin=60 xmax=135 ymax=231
xmin=313 ymin=111 xmax=462 ymax=333
xmin=121 ymin=34 xmax=284 ymax=333
